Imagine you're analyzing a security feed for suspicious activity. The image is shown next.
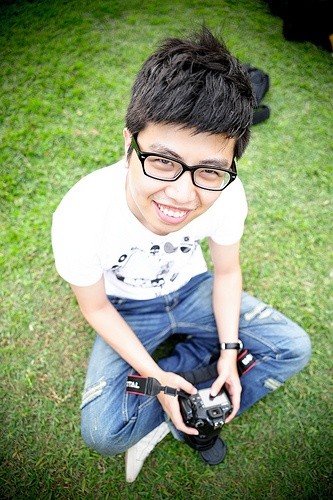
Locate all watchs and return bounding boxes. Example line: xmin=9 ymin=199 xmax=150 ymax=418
xmin=217 ymin=339 xmax=244 ymax=355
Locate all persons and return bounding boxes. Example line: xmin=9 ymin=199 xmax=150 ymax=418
xmin=49 ymin=17 xmax=312 ymax=484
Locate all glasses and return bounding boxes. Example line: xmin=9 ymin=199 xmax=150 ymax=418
xmin=128 ymin=128 xmax=237 ymax=192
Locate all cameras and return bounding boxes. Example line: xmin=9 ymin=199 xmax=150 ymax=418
xmin=179 ymin=386 xmax=232 ymax=451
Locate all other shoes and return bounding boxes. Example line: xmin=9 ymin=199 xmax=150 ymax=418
xmin=125 ymin=420 xmax=170 ymax=483
